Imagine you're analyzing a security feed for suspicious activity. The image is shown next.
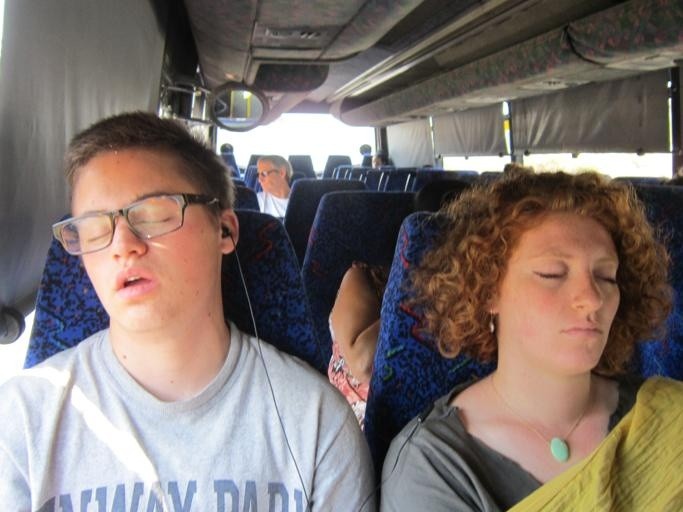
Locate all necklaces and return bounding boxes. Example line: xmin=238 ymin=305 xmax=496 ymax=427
xmin=487 ymin=364 xmax=596 ymax=464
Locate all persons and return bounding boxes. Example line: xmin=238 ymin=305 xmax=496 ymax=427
xmin=1 ymin=110 xmax=379 ymax=512
xmin=379 ymin=163 xmax=681 ymax=512
xmin=254 ymin=154 xmax=294 ymax=225
xmin=370 ymin=153 xmax=388 ymax=170
xmin=325 ymin=174 xmax=473 ymax=431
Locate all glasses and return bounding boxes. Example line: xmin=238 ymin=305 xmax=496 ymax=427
xmin=53 ymin=192 xmax=222 ymax=255
xmin=256 ymin=168 xmax=278 ymax=176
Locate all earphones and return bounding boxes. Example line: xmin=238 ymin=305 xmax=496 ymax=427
xmin=222 ymin=222 xmax=231 ymax=239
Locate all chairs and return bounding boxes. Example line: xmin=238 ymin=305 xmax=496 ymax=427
xmin=26 ymin=142 xmax=682 ymax=510
